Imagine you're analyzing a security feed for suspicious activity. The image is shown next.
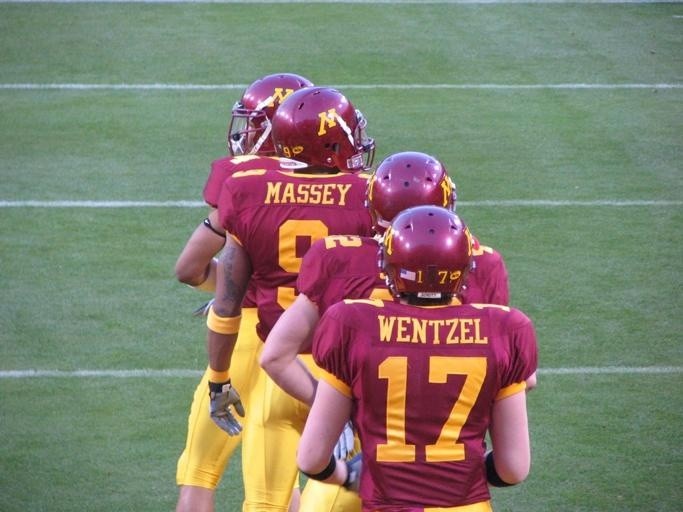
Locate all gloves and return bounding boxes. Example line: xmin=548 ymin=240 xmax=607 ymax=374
xmin=208 ymin=378 xmax=245 ymax=436
xmin=343 ymin=452 xmax=362 ymax=491
xmin=332 ymin=419 xmax=354 ymax=459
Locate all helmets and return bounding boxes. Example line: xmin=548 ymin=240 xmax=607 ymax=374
xmin=271 ymin=88 xmax=375 ymax=173
xmin=226 ymin=72 xmax=313 ymax=156
xmin=366 ymin=151 xmax=456 ymax=235
xmin=382 ymin=205 xmax=471 ymax=306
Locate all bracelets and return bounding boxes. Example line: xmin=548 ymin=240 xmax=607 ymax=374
xmin=206 ymin=366 xmax=230 ymax=383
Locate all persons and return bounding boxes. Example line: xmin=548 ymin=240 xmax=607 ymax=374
xmin=206 ymin=84 xmax=378 ymax=512
xmin=175 ymin=73 xmax=315 ymax=511
xmin=295 ymin=204 xmax=540 ymax=512
xmin=259 ymin=151 xmax=511 ymax=512
